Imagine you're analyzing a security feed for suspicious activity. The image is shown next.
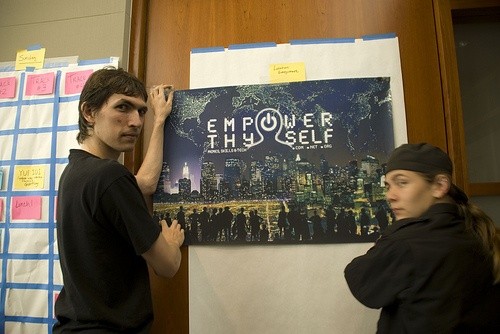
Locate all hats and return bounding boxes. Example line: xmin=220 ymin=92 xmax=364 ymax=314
xmin=385 ymin=144 xmax=468 ymax=204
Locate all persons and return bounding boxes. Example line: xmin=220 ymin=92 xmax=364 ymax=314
xmin=344 ymin=145 xmax=500 ymax=334
xmin=51 ymin=69 xmax=185 ymax=334
xmin=153 ymin=201 xmax=395 ymax=241
